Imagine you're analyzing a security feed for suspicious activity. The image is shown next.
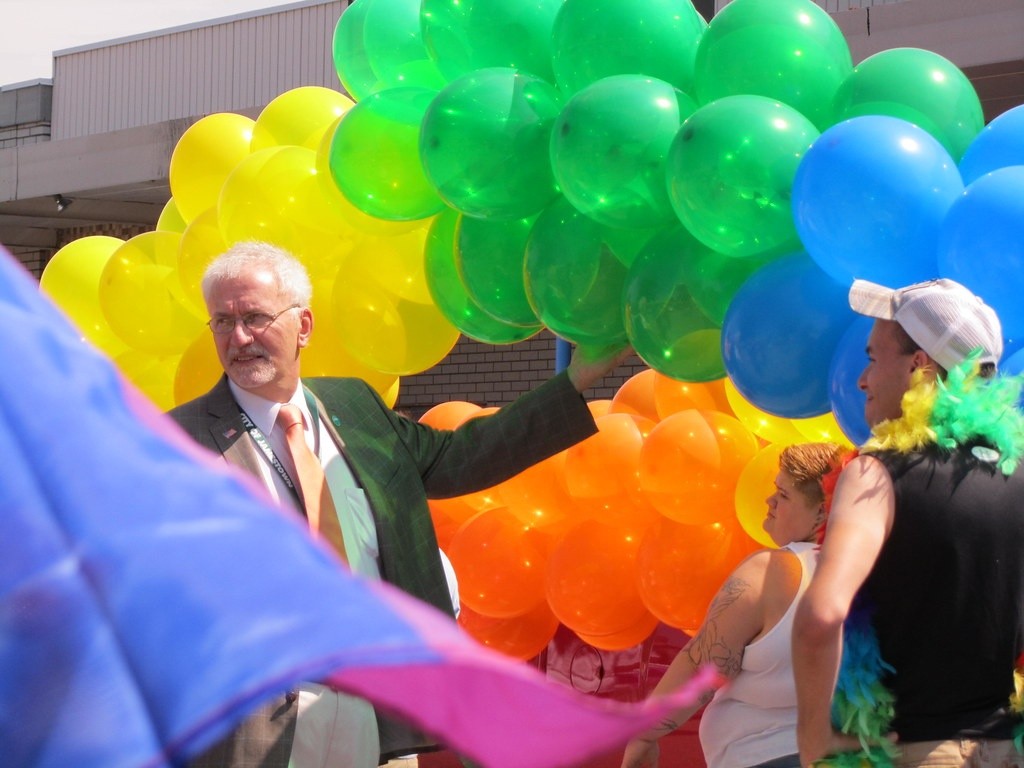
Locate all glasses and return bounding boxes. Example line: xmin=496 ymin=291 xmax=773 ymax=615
xmin=207 ymin=304 xmax=302 ymax=334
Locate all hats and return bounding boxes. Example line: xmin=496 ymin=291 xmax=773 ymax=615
xmin=849 ymin=278 xmax=1004 ymax=379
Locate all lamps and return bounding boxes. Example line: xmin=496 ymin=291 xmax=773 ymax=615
xmin=54 ymin=194 xmax=72 ymax=213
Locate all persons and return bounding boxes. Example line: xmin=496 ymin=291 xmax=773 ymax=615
xmin=791 ymin=278 xmax=1024 ymax=768
xmin=620 ymin=442 xmax=854 ymax=768
xmin=160 ymin=243 xmax=633 ymax=767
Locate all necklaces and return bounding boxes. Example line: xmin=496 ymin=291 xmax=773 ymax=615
xmin=812 ymin=345 xmax=1023 ymax=768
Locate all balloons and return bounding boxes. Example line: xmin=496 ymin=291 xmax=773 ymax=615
xmin=38 ymin=1 xmax=1024 ymax=666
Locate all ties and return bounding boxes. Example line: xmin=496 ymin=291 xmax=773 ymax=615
xmin=277 ymin=403 xmax=349 ymax=569
